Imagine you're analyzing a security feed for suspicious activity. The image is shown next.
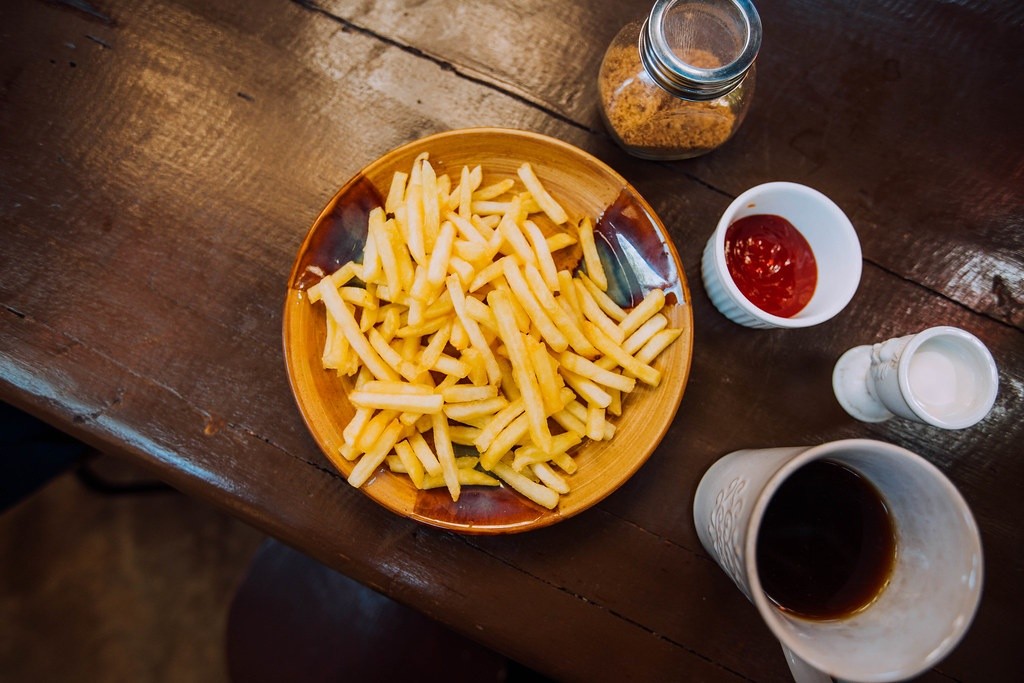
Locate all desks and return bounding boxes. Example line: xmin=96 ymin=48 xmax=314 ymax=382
xmin=0 ymin=0 xmax=1024 ymax=683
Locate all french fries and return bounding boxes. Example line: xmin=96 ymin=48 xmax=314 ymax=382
xmin=305 ymin=150 xmax=686 ymax=508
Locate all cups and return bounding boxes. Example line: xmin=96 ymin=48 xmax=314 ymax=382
xmin=832 ymin=324 xmax=999 ymax=430
xmin=692 ymin=436 xmax=985 ymax=682
xmin=700 ymin=180 xmax=863 ymax=331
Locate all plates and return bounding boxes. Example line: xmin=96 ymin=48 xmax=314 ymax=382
xmin=281 ymin=128 xmax=694 ymax=536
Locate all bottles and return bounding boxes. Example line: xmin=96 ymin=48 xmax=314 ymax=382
xmin=593 ymin=0 xmax=762 ymax=162
